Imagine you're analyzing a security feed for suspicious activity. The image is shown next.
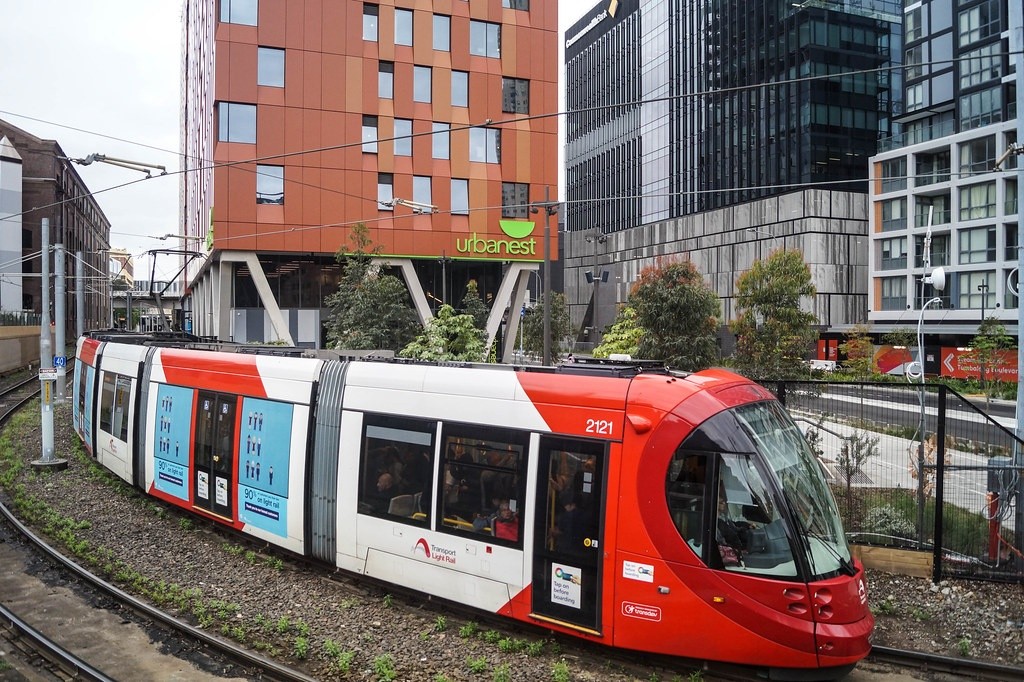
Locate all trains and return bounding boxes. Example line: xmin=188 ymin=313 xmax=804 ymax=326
xmin=72 ymin=327 xmax=877 ymax=682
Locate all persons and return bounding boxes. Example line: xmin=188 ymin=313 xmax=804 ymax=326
xmin=480 ymin=452 xmax=514 ymax=516
xmin=369 ymin=443 xmax=425 ymax=514
xmin=495 ymin=501 xmax=518 ymax=541
xmin=549 ymin=496 xmax=588 ymax=563
xmin=445 ymin=445 xmax=473 ymax=504
xmin=549 ymin=461 xmax=571 ymax=497
xmin=719 ymin=498 xmax=756 ymax=553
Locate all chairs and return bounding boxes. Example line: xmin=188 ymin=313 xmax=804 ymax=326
xmin=388 ymin=492 xmax=424 ymax=520
xmin=491 ymin=517 xmax=497 ymax=537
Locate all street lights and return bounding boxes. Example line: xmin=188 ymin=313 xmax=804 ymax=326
xmin=585 ymin=223 xmax=609 ymax=347
xmin=530 ymin=185 xmax=560 ymax=367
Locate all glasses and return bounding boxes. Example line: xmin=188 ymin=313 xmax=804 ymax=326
xmin=720 ymin=502 xmax=725 ymax=505
